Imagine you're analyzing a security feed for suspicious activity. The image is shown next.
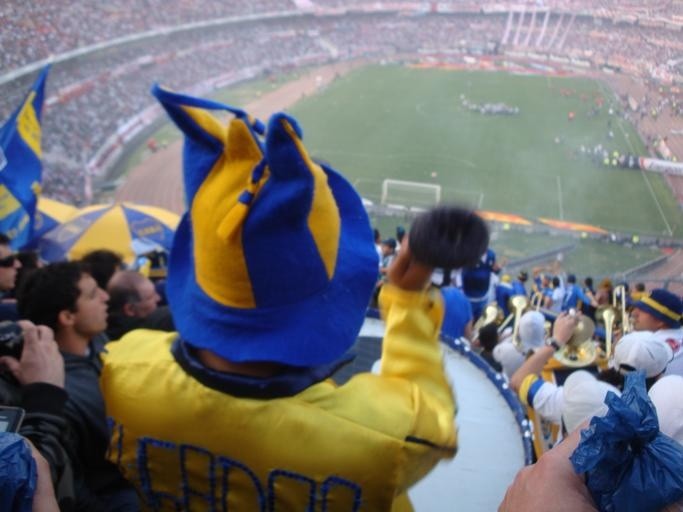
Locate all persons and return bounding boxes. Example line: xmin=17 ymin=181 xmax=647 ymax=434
xmin=1 ymin=1 xmax=683 ymax=204
xmin=371 ymin=225 xmax=682 ymax=510
xmin=546 ymin=0 xmax=681 ymax=179
xmin=1 ymin=234 xmax=175 ymax=511
xmin=102 ymin=80 xmax=459 ymax=511
xmin=457 ymin=92 xmax=521 ymax=118
xmin=338 ymin=0 xmax=545 ymax=75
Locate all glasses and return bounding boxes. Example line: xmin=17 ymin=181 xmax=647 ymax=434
xmin=1 ymin=255 xmax=14 ymax=268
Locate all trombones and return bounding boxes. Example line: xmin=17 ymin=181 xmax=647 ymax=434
xmin=510 ymin=292 xmax=542 ymax=346
xmin=469 ymin=303 xmax=514 ymax=352
xmin=594 ymin=283 xmax=629 ymax=362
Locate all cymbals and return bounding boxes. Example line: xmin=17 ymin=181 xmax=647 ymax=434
xmin=564 ymin=313 xmax=595 ymax=347
xmin=553 ymin=341 xmax=599 ymax=368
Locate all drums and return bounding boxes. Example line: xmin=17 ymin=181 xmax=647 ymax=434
xmin=327 ymin=309 xmax=540 ymax=512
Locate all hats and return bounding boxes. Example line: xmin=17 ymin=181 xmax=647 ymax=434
xmin=635 ymin=288 xmax=683 ymax=327
xmin=613 ymin=330 xmax=674 ymax=378
xmin=519 ymin=311 xmax=545 ymax=348
xmin=563 ymin=370 xmax=602 ymax=435
xmin=150 ymin=84 xmax=380 ymax=368
